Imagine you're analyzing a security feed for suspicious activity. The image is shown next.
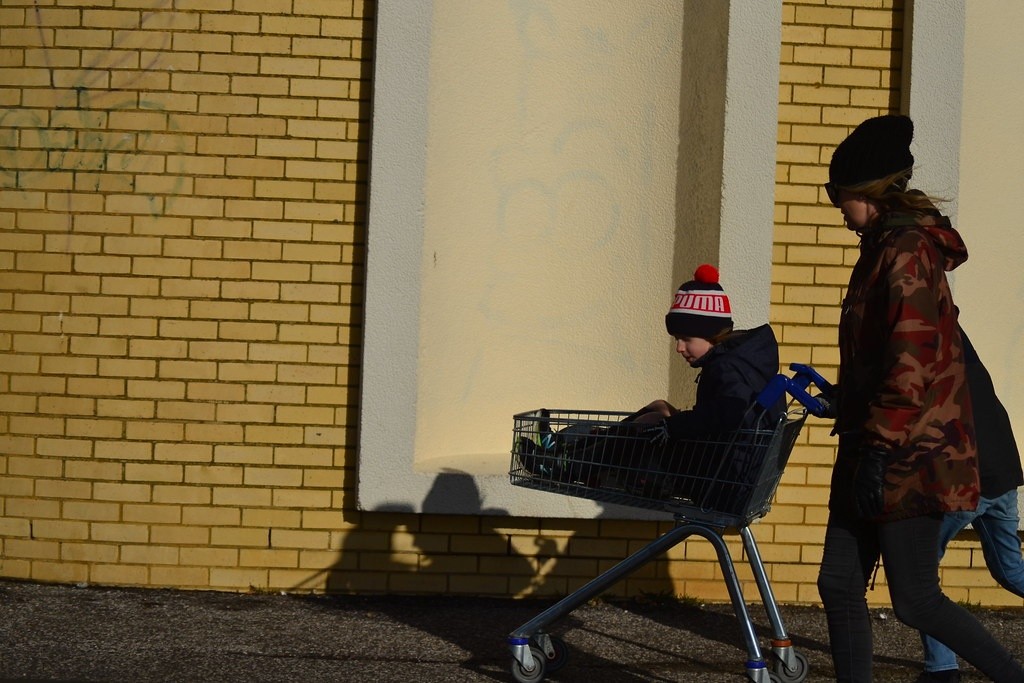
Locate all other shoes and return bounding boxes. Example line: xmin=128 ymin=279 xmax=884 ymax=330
xmin=911 ymin=669 xmax=959 ymax=683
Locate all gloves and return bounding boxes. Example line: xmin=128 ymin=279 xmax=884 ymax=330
xmin=846 ymin=443 xmax=892 ymax=515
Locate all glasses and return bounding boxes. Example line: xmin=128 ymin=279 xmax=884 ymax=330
xmin=824 ymin=182 xmax=839 ymax=208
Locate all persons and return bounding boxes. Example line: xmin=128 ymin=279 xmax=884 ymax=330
xmin=804 ymin=115 xmax=1024 ymax=683
xmin=518 ymin=264 xmax=787 ymax=517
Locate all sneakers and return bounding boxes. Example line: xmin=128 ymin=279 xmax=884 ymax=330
xmin=516 ymin=437 xmax=579 ymax=485
xmin=534 ymin=409 xmax=567 ymax=456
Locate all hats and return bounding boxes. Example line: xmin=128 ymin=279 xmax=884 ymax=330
xmin=829 ymin=115 xmax=914 ymax=193
xmin=666 ymin=264 xmax=733 ymax=335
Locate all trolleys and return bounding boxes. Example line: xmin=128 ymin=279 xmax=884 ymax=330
xmin=503 ymin=362 xmax=832 ymax=683
